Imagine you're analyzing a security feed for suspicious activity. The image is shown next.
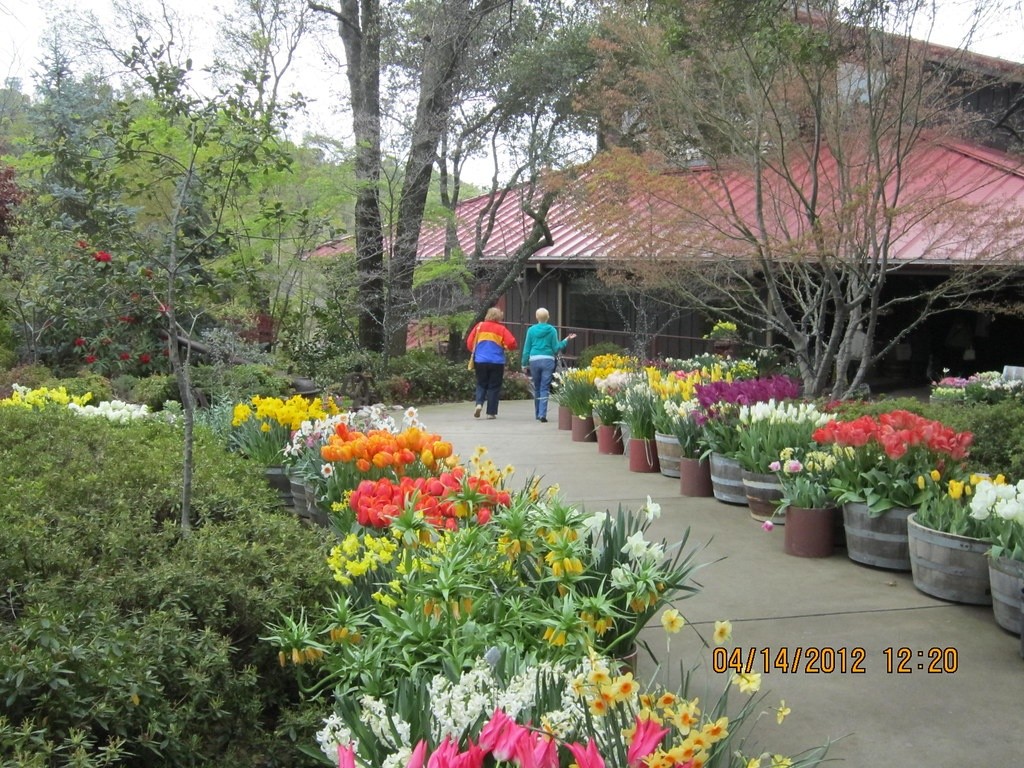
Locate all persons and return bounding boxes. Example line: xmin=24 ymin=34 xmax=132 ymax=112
xmin=521 ymin=308 xmax=577 ymax=422
xmin=467 ymin=308 xmax=518 ymax=419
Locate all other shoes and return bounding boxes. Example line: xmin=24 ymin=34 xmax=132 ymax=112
xmin=473 ymin=404 xmax=482 ymax=418
xmin=487 ymin=414 xmax=496 ymax=419
xmin=536 ymin=416 xmax=547 ymax=422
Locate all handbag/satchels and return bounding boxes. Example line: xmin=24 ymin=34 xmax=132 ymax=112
xmin=468 ymin=353 xmax=475 ymax=370
xmin=555 ymin=344 xmax=568 ymax=374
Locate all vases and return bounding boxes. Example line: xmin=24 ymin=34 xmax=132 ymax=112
xmin=259 ymin=466 xmax=331 ymax=529
xmin=929 ymin=394 xmax=968 ymax=405
xmin=608 ymin=641 xmax=638 ymax=681
xmin=713 ymin=338 xmax=741 ymax=360
xmin=558 ymin=405 xmax=786 ymax=525
xmin=784 ymin=502 xmax=1024 ymax=635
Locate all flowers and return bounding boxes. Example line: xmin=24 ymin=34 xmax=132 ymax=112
xmin=702 ymin=320 xmax=738 ymax=341
xmin=541 ymin=352 xmax=837 ymax=472
xmin=759 ymin=409 xmax=1023 ymax=560
xmin=927 ymin=366 xmax=1024 ymax=400
xmin=231 ymin=391 xmax=453 ymax=485
xmin=322 ymin=468 xmax=857 ymax=768
